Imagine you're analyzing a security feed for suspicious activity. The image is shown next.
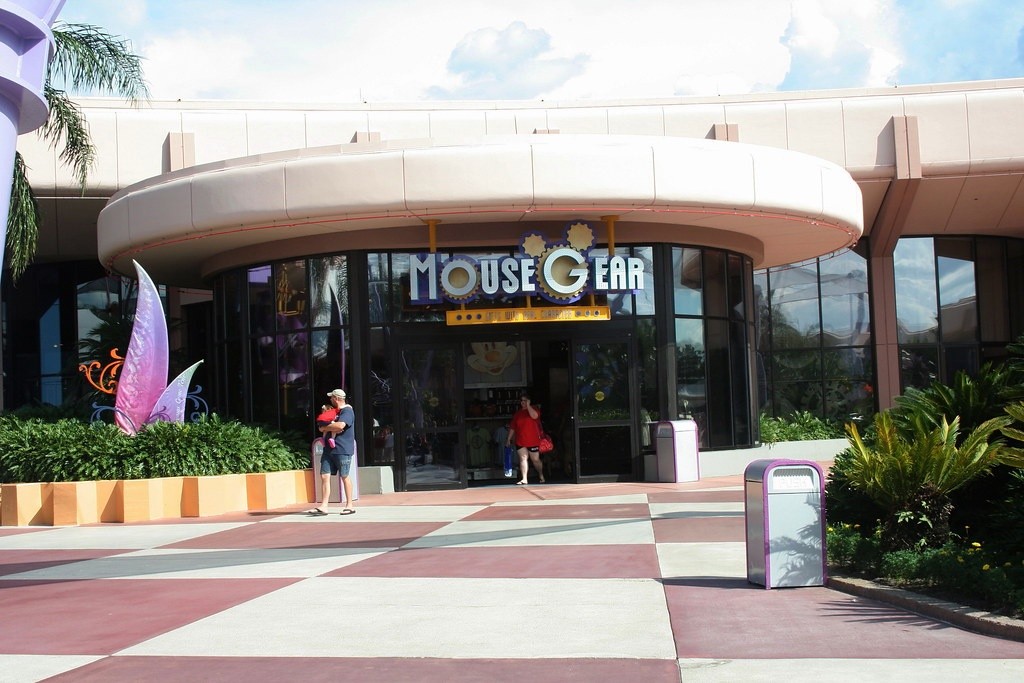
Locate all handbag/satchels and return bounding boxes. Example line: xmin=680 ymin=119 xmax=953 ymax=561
xmin=504 ymin=447 xmax=514 ymax=476
xmin=539 ymin=433 xmax=553 ymax=454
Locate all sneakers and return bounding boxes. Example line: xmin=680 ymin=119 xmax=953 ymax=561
xmin=318 ymin=437 xmax=326 ymax=447
xmin=328 ymin=438 xmax=335 ymax=448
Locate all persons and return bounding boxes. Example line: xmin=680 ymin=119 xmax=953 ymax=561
xmin=504 ymin=392 xmax=546 ymax=486
xmin=316 ymin=403 xmax=353 ymax=450
xmin=307 ymin=388 xmax=356 ymax=516
xmin=371 ymin=415 xmax=455 ymax=464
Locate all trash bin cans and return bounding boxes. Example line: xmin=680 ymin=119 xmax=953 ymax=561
xmin=311 ymin=437 xmax=359 ymax=503
xmin=743 ymin=458 xmax=830 ymax=589
xmin=655 ymin=420 xmax=701 ymax=484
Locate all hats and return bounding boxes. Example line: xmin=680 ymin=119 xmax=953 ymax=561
xmin=327 ymin=388 xmax=346 ymax=399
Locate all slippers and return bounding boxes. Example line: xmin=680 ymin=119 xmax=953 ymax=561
xmin=340 ymin=508 xmax=355 ymax=515
xmin=515 ymin=480 xmax=528 ymax=485
xmin=539 ymin=479 xmax=546 ymax=483
xmin=307 ymin=507 xmax=329 ymax=515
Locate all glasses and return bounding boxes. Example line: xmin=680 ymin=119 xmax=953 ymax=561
xmin=520 ymin=399 xmax=525 ymax=402
